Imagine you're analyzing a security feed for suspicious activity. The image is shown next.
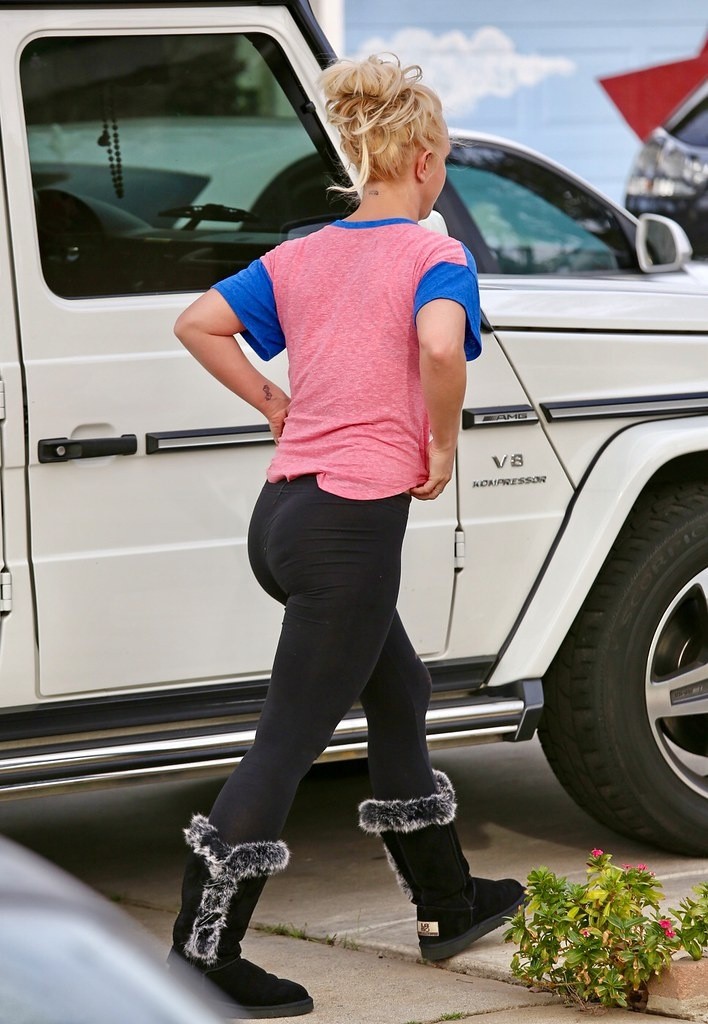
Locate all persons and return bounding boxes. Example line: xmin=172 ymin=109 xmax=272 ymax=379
xmin=164 ymin=54 xmax=526 ymax=1020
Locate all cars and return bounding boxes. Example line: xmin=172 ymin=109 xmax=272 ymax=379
xmin=24 ymin=119 xmax=708 ymax=280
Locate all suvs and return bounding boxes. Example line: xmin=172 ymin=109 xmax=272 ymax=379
xmin=0 ymin=0 xmax=708 ymax=860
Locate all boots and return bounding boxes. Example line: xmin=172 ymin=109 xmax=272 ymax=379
xmin=166 ymin=814 xmax=315 ymax=1018
xmin=358 ymin=767 xmax=528 ymax=961
